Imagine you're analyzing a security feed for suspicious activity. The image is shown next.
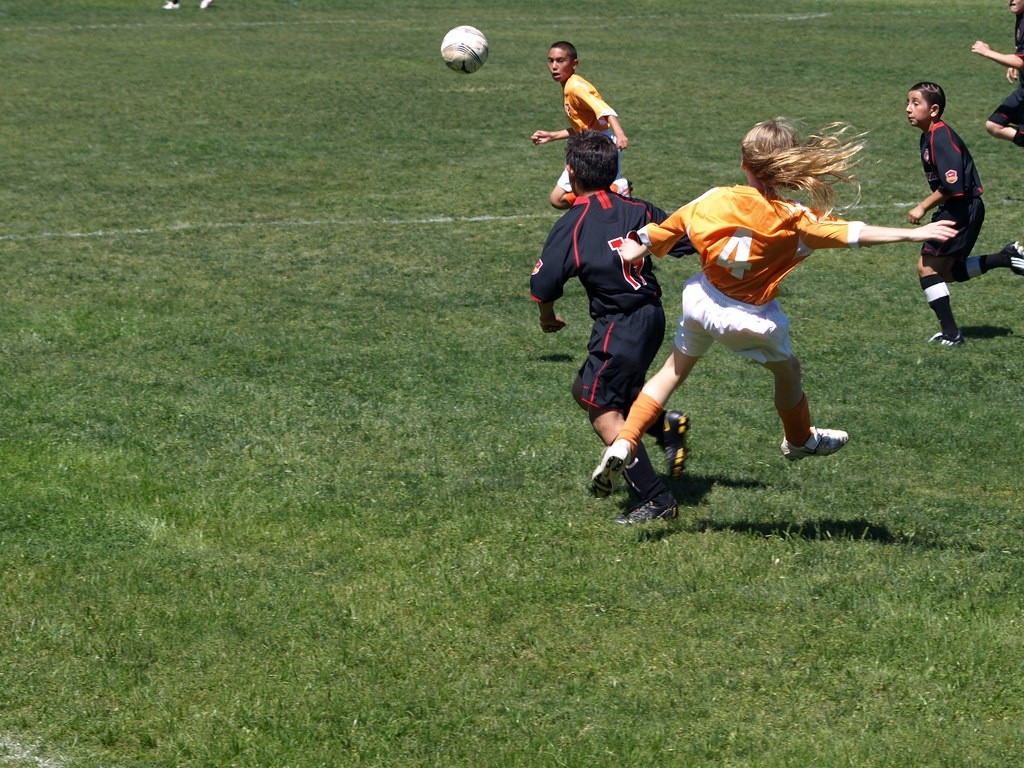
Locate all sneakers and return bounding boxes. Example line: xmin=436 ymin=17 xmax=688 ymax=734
xmin=615 ymin=499 xmax=680 ymax=528
xmin=614 ymin=178 xmax=633 ymax=199
xmin=591 ymin=439 xmax=639 ymax=498
xmin=162 ymin=1 xmax=180 ymax=9
xmin=781 ymin=427 xmax=849 ymax=462
xmin=654 ymin=409 xmax=690 ymax=482
xmin=201 ymin=0 xmax=214 ymax=9
xmin=926 ymin=330 xmax=964 ymax=347
xmin=1001 ymin=240 xmax=1024 ymax=277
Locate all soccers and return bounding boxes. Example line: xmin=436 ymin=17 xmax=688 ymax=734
xmin=440 ymin=26 xmax=489 ymax=74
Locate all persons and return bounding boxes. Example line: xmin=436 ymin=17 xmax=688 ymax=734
xmin=906 ymin=83 xmax=1024 ymax=346
xmin=971 ymin=0 xmax=1024 ymax=147
xmin=162 ymin=0 xmax=212 ymax=10
xmin=531 ymin=42 xmax=634 ymax=210
xmin=592 ymin=121 xmax=959 ymax=498
xmin=530 ymin=132 xmax=699 ymax=527
